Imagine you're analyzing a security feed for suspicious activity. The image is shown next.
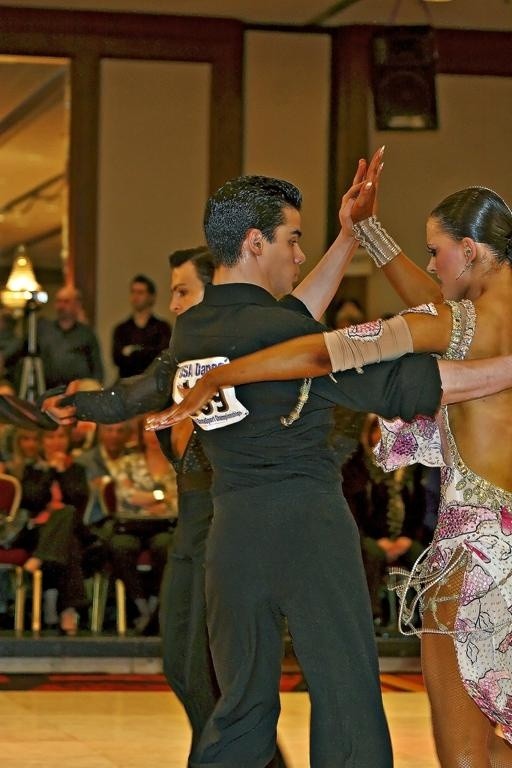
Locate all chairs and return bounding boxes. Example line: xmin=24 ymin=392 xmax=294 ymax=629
xmin=0 ymin=473 xmax=152 ymax=637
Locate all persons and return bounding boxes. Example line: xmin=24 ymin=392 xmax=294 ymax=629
xmin=1 ymin=275 xmax=441 ymax=634
xmin=41 ymin=145 xmax=511 ymax=767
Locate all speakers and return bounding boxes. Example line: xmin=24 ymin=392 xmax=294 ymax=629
xmin=369 ymin=25 xmax=436 ymax=130
